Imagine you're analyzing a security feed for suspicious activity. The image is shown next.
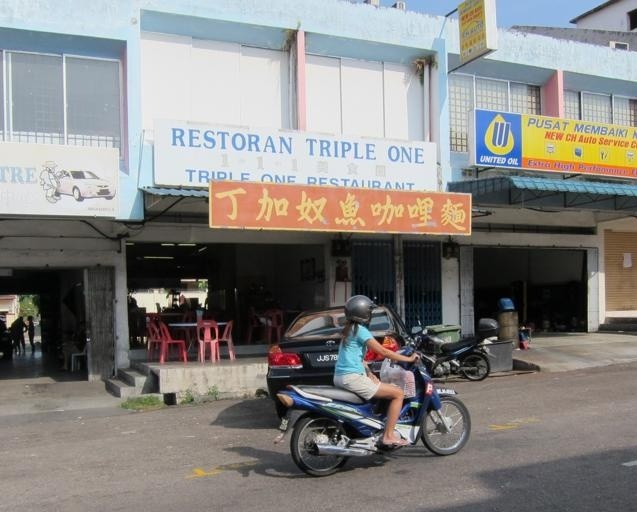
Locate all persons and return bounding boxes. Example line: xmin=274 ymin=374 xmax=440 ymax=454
xmin=26 ymin=315 xmax=36 ymax=352
xmin=8 ymin=323 xmax=18 ymax=353
xmin=330 ymin=294 xmax=420 ymax=446
xmin=12 ymin=317 xmax=28 ymax=352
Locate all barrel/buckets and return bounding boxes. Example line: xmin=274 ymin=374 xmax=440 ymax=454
xmin=498 ymin=310 xmax=520 ymax=348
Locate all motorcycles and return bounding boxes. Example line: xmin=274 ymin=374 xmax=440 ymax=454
xmin=407 ymin=315 xmax=500 ymax=383
xmin=270 ymin=316 xmax=472 ymax=478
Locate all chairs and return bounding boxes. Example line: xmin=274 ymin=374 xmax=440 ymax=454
xmin=72 ymin=344 xmax=87 ymax=372
xmin=146 ymin=307 xmax=236 ymax=364
xmin=246 ymin=307 xmax=265 ymax=344
xmin=265 ymin=308 xmax=285 ymax=345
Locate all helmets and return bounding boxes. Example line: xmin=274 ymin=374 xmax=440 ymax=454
xmin=344 ymin=293 xmax=378 ymax=326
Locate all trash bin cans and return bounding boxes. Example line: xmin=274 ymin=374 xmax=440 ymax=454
xmin=479 ymin=298 xmax=520 ymax=373
xmin=427 ymin=324 xmax=461 ymax=343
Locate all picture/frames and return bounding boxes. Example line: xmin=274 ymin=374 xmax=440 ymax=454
xmin=299 ymin=257 xmax=316 ymax=282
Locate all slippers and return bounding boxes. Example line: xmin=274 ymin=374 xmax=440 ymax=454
xmin=383 ymin=438 xmax=409 ymax=446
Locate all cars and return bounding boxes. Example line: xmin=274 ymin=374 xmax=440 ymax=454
xmin=0 ymin=318 xmax=13 ymax=362
xmin=55 ymin=168 xmax=116 ymax=203
xmin=263 ymin=301 xmax=423 ymax=422
